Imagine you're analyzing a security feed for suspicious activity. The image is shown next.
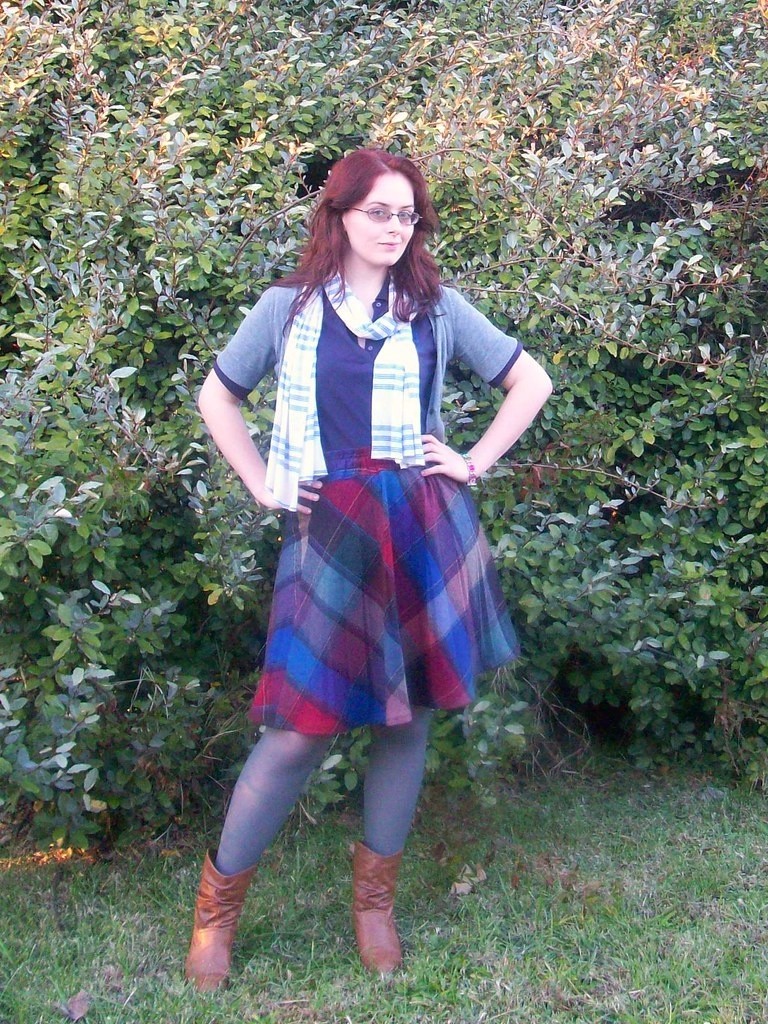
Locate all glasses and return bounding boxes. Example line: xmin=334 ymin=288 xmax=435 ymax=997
xmin=348 ymin=207 xmax=424 ymax=226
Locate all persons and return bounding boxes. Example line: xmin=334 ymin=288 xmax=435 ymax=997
xmin=187 ymin=148 xmax=553 ymax=994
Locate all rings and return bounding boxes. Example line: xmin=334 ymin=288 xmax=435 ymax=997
xmin=306 ymin=480 xmax=312 ymax=487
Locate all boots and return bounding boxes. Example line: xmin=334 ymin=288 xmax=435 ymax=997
xmin=184 ymin=847 xmax=258 ymax=994
xmin=351 ymin=838 xmax=402 ymax=976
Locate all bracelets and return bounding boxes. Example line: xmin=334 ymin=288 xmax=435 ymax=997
xmin=462 ymin=454 xmax=477 ymax=486
